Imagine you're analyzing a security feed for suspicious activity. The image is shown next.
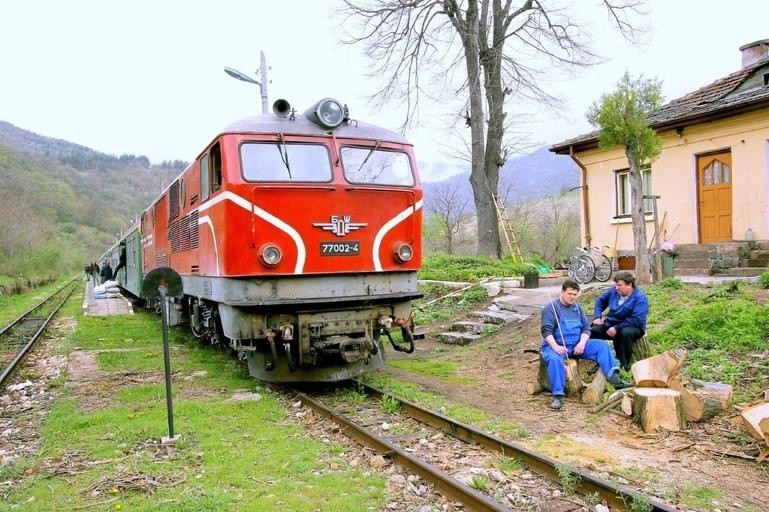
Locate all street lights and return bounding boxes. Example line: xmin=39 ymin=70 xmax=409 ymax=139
xmin=224 ymin=50 xmax=268 ymax=115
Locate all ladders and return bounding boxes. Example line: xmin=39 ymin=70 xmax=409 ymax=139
xmin=491 ymin=192 xmax=524 ymax=264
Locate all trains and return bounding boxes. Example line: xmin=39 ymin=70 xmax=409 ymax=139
xmin=97 ymin=97 xmax=425 ymax=383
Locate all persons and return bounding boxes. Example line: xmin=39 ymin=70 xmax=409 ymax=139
xmin=590 ymin=271 xmax=650 ymax=373
xmin=84 ymin=259 xmax=113 ymax=287
xmin=110 ymin=242 xmax=126 ymax=281
xmin=541 ymin=279 xmax=634 ymax=408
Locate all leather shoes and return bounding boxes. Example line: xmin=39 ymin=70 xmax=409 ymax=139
xmin=551 ymin=399 xmax=564 ymax=409
xmin=613 ymin=380 xmax=634 ymax=390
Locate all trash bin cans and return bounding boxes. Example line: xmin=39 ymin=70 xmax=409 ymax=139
xmin=652 ymin=252 xmax=674 ymax=280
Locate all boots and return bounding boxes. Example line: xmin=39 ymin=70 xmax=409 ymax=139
xmin=587 ymin=364 xmax=598 ymax=375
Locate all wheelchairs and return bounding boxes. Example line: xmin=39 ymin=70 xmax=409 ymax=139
xmin=568 ymin=245 xmax=612 ymax=284
